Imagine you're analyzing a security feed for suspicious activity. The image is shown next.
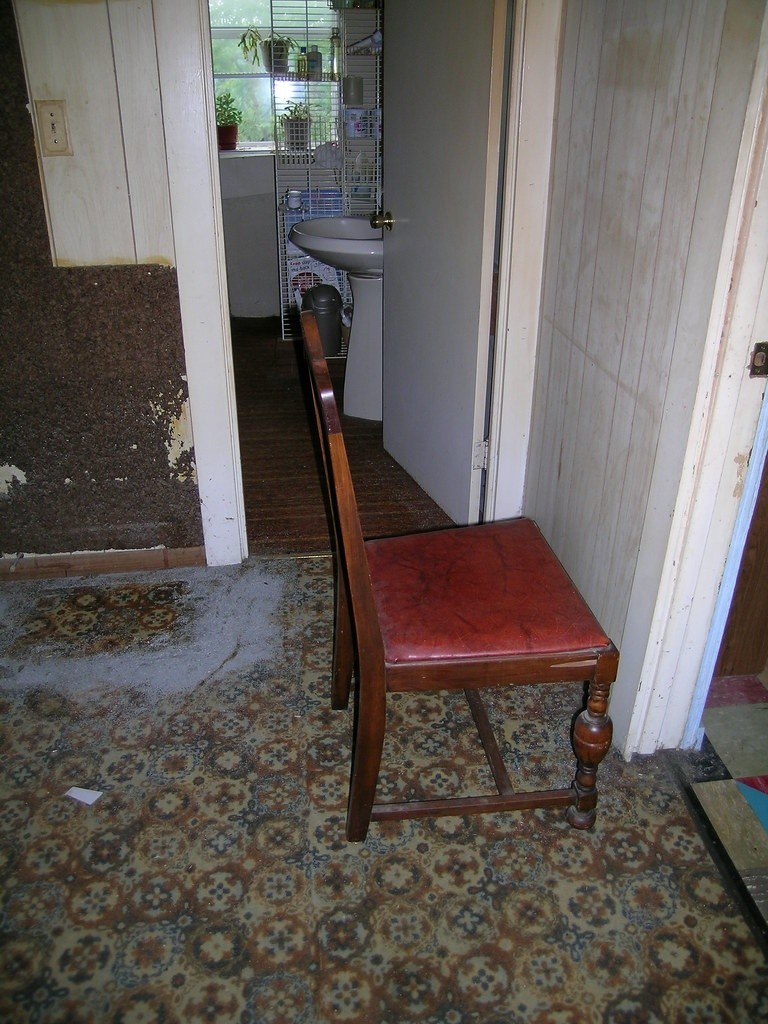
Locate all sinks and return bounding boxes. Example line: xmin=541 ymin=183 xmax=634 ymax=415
xmin=287 ymin=216 xmax=383 ymax=276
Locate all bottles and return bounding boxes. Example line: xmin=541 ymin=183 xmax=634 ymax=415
xmin=307 ymin=45 xmax=322 ymax=81
xmin=297 ymin=47 xmax=308 ymax=81
xmin=329 ymin=27 xmax=341 ymax=82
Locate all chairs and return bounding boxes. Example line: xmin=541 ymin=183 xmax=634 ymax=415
xmin=289 ymin=296 xmax=619 ymax=844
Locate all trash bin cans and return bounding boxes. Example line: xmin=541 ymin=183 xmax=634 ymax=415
xmin=301 ymin=284 xmax=343 ymax=357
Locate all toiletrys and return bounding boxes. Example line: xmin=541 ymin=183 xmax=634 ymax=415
xmin=297 ymin=47 xmax=307 ymax=81
xmin=330 ymin=27 xmax=341 ymax=81
xmin=307 ymin=45 xmax=322 ymax=81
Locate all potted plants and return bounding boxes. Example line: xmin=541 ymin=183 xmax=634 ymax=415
xmin=216 ymin=93 xmax=242 ymax=150
xmin=276 ymin=100 xmax=322 ymax=149
xmin=237 ymin=24 xmax=301 ymax=74
